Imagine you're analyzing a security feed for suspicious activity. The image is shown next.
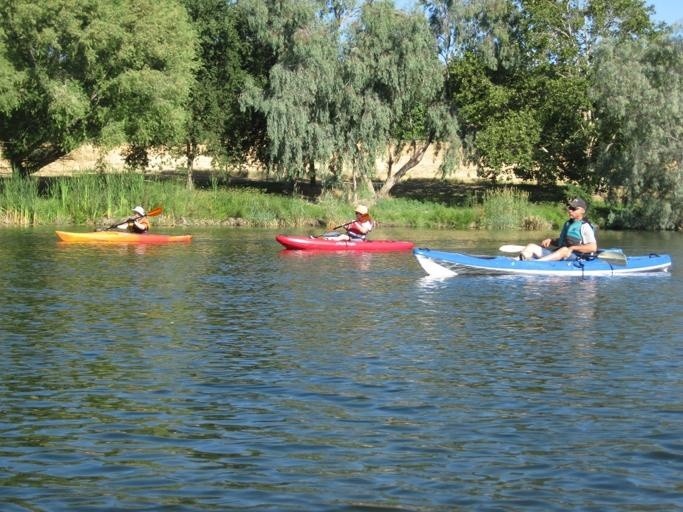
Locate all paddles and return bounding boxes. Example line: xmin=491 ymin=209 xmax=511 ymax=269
xmin=106 ymin=207 xmax=163 ymax=232
xmin=498 ymin=244 xmax=626 ymax=265
xmin=330 ymin=213 xmax=369 ymax=231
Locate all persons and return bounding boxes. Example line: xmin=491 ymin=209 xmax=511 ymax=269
xmin=108 ymin=205 xmax=150 ymax=234
xmin=309 ymin=204 xmax=374 ymax=243
xmin=514 ymin=197 xmax=597 ymax=262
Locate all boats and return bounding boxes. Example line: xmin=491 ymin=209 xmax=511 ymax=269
xmin=408 ymin=240 xmax=675 ymax=277
xmin=54 ymin=229 xmax=194 ymax=243
xmin=274 ymin=233 xmax=416 ymax=253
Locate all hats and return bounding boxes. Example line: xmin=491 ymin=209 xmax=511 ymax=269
xmin=132 ymin=205 xmax=145 ymax=217
xmin=567 ymin=197 xmax=586 ymax=208
xmin=354 ymin=204 xmax=368 ymax=215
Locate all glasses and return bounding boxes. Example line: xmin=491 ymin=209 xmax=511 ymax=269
xmin=568 ymin=205 xmax=580 ymax=210
